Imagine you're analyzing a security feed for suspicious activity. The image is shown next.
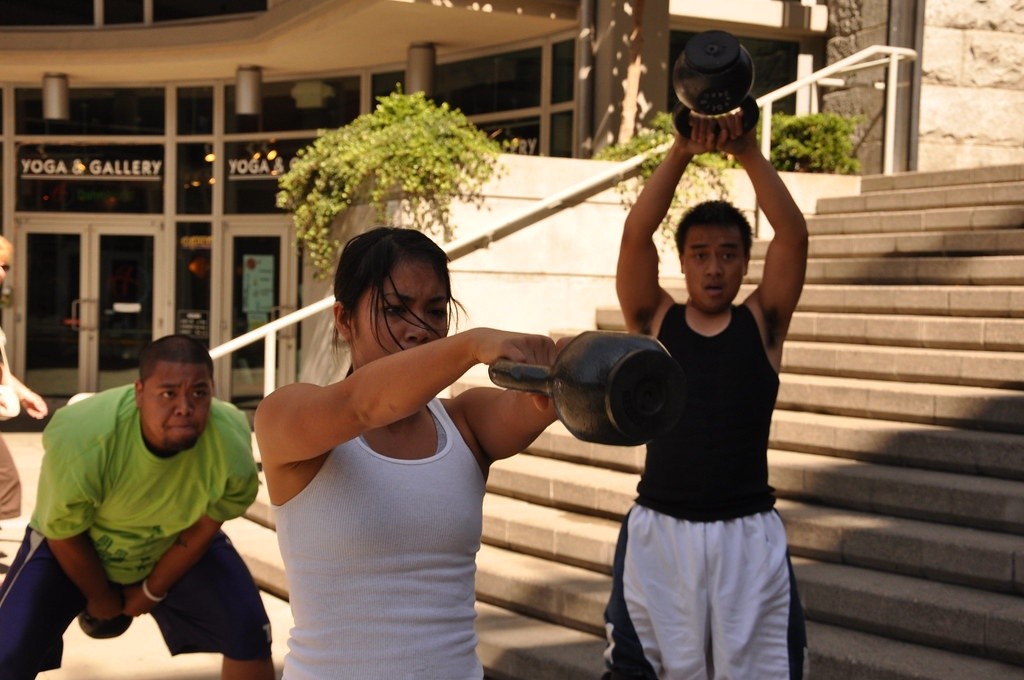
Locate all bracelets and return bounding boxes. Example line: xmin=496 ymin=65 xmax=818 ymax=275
xmin=142 ymin=583 xmax=162 ymax=601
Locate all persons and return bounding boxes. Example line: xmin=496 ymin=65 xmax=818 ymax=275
xmin=0 ymin=236 xmax=49 ymax=519
xmin=255 ymin=224 xmax=576 ymax=679
xmin=1 ymin=333 xmax=276 ymax=680
xmin=603 ymin=104 xmax=811 ymax=680
